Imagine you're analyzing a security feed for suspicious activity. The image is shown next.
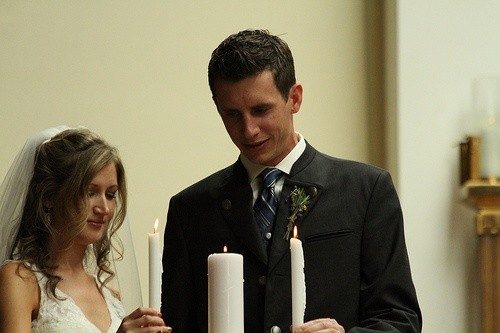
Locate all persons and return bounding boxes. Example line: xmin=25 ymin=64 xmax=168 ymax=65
xmin=161 ymin=31 xmax=423 ymax=333
xmin=0 ymin=127 xmax=172 ymax=333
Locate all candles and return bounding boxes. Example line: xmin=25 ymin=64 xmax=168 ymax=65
xmin=147 ymin=218 xmax=307 ymax=333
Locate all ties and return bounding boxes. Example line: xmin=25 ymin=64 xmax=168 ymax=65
xmin=253 ymin=167 xmax=284 ymax=232
